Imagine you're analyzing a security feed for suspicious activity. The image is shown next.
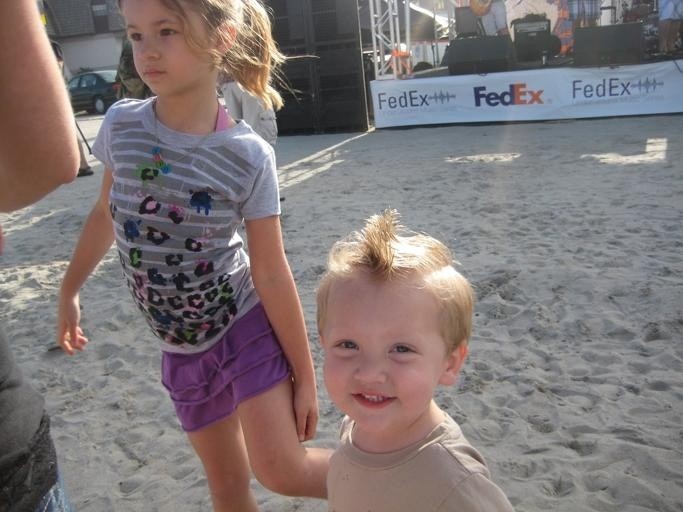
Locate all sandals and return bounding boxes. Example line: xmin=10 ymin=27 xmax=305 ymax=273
xmin=77 ymin=167 xmax=94 ymax=177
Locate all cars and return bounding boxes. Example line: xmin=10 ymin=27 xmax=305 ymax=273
xmin=66 ymin=71 xmax=117 ymax=113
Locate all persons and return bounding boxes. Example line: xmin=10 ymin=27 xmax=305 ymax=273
xmin=54 ymin=0 xmax=338 ymax=512
xmin=36 ymin=0 xmax=94 ymax=176
xmin=315 ymin=206 xmax=515 ymax=512
xmin=546 ymin=0 xmax=560 ymax=33
xmin=219 ymin=69 xmax=286 ymax=202
xmin=480 ymin=0 xmax=513 ymax=71
xmin=0 ymin=0 xmax=84 ymax=512
